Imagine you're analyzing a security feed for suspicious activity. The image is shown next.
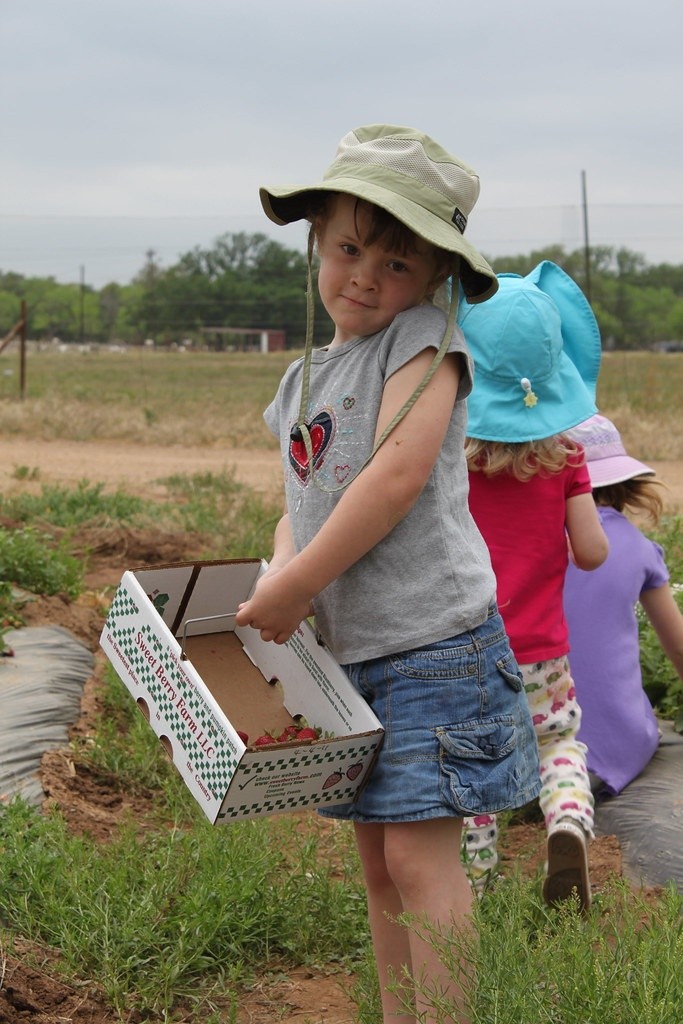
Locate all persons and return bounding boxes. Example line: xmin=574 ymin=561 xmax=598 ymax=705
xmin=235 ymin=122 xmax=538 ymax=1024
xmin=461 ymin=258 xmax=610 ymax=916
xmin=560 ymin=411 xmax=683 ymax=804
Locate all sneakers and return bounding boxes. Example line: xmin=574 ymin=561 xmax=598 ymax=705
xmin=541 ymin=816 xmax=592 ymax=925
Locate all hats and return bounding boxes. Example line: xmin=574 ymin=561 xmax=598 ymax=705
xmin=561 ymin=414 xmax=658 ymax=490
xmin=258 ymin=122 xmax=500 ymax=305
xmin=440 ymin=258 xmax=603 ymax=443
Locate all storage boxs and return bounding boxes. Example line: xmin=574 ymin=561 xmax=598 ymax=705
xmin=100 ymin=556 xmax=387 ymax=828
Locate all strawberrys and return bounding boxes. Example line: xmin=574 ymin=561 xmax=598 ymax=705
xmin=234 ymin=724 xmax=323 ymax=748
